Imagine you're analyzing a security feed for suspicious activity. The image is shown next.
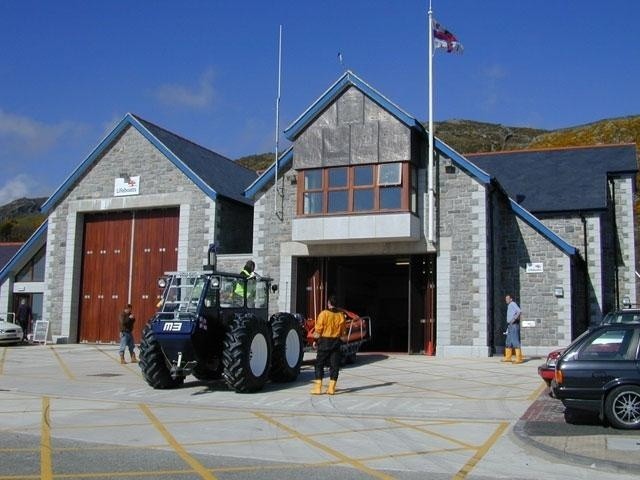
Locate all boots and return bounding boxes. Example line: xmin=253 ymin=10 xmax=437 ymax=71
xmin=500 ymin=348 xmax=512 ymax=362
xmin=512 ymin=348 xmax=523 ymax=364
xmin=119 ymin=351 xmax=139 ymax=364
xmin=325 ymin=379 xmax=337 ymax=395
xmin=310 ymin=379 xmax=322 ymax=394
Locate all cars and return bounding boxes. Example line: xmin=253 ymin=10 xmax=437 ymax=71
xmin=538 ymin=309 xmax=640 ymax=429
xmin=0 ymin=318 xmax=24 ymax=343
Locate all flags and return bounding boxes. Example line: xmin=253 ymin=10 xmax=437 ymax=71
xmin=430 ymin=19 xmax=465 ymax=56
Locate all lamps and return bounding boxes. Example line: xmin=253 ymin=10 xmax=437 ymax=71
xmin=290 ymin=174 xmax=296 ymax=184
xmin=444 ymin=158 xmax=452 ymax=167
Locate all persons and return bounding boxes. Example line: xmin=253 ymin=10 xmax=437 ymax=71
xmin=119 ymin=303 xmax=139 ymax=364
xmin=499 ymin=292 xmax=524 ymax=364
xmin=232 ymin=260 xmax=257 ymax=308
xmin=15 ymin=300 xmax=34 ymax=340
xmin=311 ymin=295 xmax=348 ymax=395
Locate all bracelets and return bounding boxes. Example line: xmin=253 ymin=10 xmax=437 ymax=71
xmin=514 ymin=318 xmax=517 ymax=320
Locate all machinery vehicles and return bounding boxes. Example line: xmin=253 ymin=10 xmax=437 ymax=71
xmin=138 ymin=244 xmax=303 ymax=393
